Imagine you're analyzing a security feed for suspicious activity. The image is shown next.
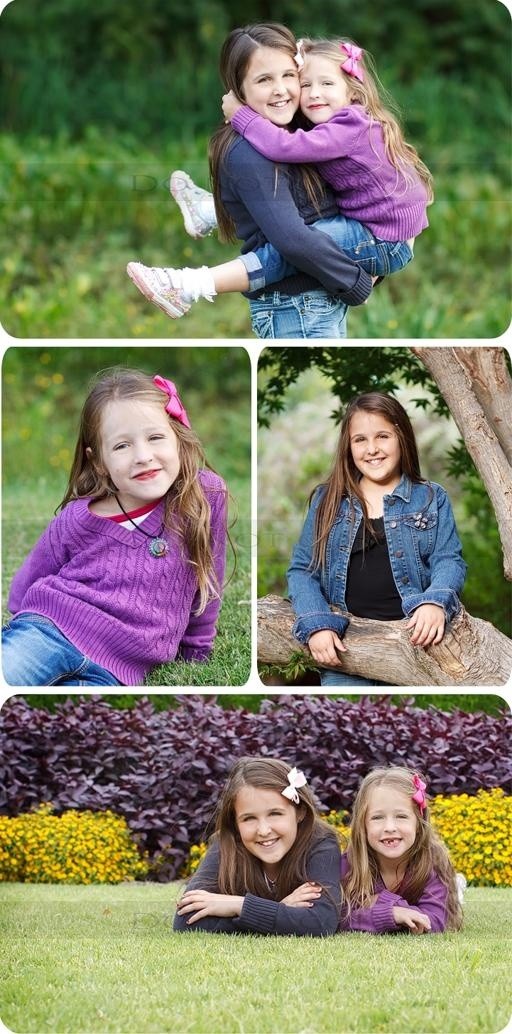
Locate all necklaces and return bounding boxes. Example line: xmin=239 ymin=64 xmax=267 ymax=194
xmin=263 ymin=868 xmax=280 ymax=893
xmin=113 ymin=490 xmax=170 ymax=559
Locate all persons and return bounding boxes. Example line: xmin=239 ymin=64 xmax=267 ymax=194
xmin=2 ymin=364 xmax=230 ymax=686
xmin=172 ymin=754 xmax=342 ymax=937
xmin=284 ymin=389 xmax=469 ymax=686
xmin=204 ymin=21 xmax=386 ymax=338
xmin=125 ymin=35 xmax=435 ymax=321
xmin=340 ymin=765 xmax=468 ymax=934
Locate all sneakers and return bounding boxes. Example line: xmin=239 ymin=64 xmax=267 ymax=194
xmin=125 ymin=260 xmax=190 ymax=317
xmin=170 ymin=170 xmax=215 ymax=240
